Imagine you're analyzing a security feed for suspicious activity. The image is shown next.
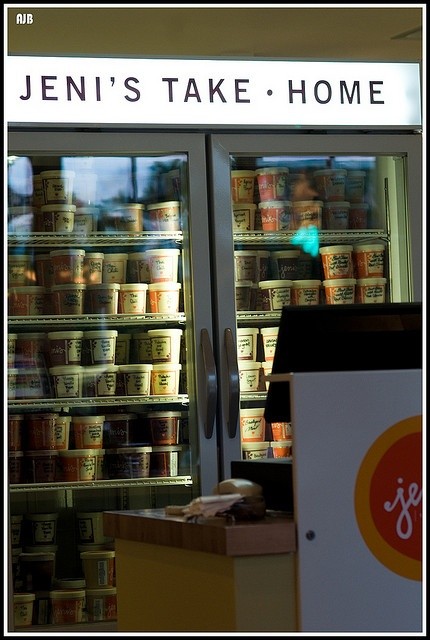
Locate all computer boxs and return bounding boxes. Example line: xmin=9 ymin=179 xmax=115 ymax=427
xmin=230 ymin=457 xmax=293 ymax=520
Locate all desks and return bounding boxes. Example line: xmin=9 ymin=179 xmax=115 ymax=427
xmin=102 ymin=506 xmax=300 ymax=631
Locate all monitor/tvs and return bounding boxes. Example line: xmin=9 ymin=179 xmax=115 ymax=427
xmin=264 ymin=299 xmax=422 ymax=423
